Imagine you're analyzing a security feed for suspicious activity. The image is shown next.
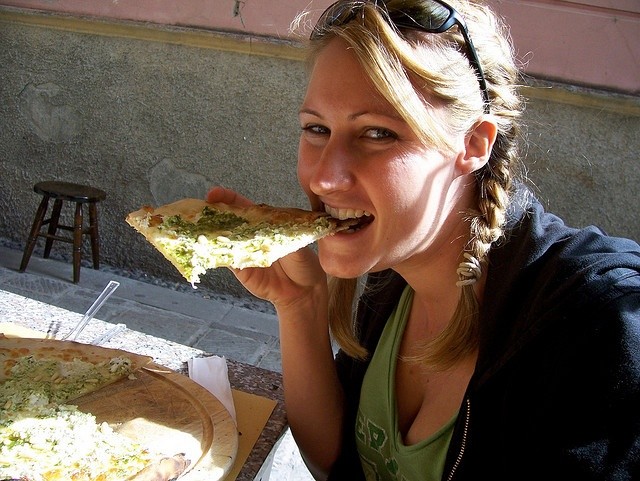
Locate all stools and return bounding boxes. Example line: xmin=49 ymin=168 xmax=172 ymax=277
xmin=19 ymin=181 xmax=107 ymax=285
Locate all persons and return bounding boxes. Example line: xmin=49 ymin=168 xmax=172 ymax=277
xmin=206 ymin=1 xmax=639 ymax=479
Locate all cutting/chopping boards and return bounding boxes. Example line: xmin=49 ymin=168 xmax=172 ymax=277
xmin=1 ymin=338 xmax=238 ymax=481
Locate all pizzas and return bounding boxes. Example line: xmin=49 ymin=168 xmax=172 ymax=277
xmin=1 ymin=332 xmax=192 ymax=481
xmin=125 ymin=198 xmax=358 ymax=289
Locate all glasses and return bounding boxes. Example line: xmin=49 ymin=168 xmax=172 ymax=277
xmin=307 ymin=1 xmax=491 ymax=113
